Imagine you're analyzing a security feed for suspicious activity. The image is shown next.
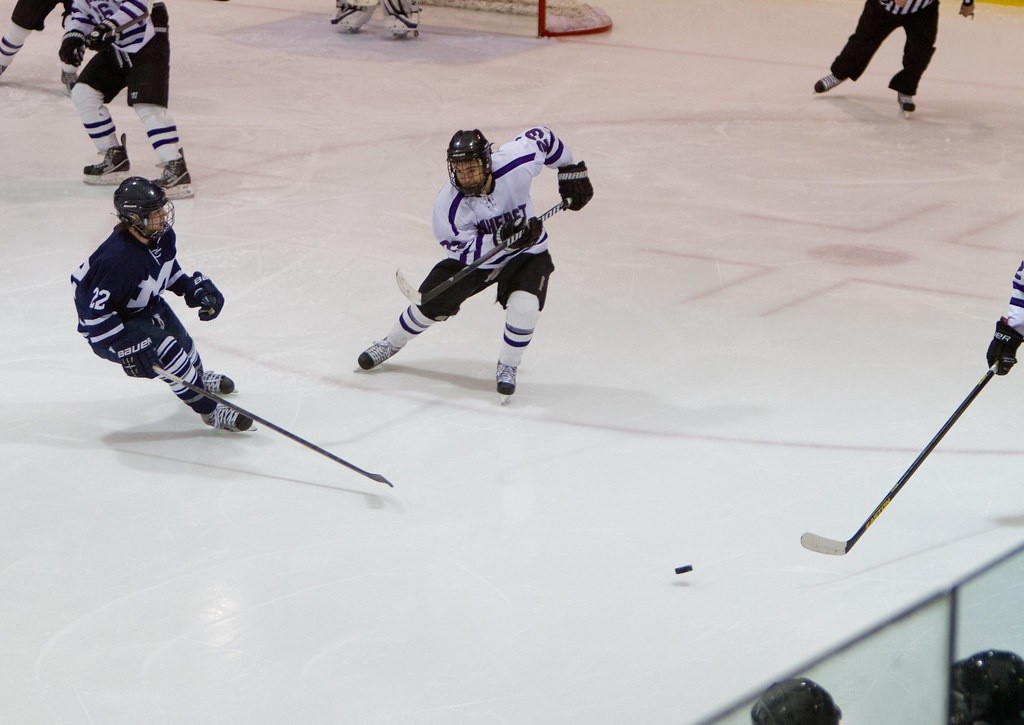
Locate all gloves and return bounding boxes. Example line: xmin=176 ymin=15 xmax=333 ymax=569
xmin=986 ymin=316 xmax=1024 ymax=375
xmin=85 ymin=17 xmax=124 ymax=49
xmin=184 ymin=271 xmax=225 ymax=321
xmin=493 ymin=217 xmax=542 ymax=253
xmin=59 ymin=29 xmax=86 ymax=67
xmin=110 ymin=329 xmax=165 ymax=379
xmin=558 ymin=160 xmax=593 ymax=211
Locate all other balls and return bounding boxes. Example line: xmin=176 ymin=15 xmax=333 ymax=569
xmin=674 ymin=564 xmax=694 ymax=574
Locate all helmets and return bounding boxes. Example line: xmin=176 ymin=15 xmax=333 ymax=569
xmin=113 ymin=176 xmax=175 ymax=240
xmin=447 ymin=129 xmax=495 ymax=197
xmin=752 ymin=678 xmax=841 ymax=725
xmin=952 ymin=648 xmax=1024 ymax=725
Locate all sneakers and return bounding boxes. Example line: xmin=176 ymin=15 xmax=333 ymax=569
xmin=496 ymin=359 xmax=517 ymax=404
xmin=354 ymin=335 xmax=400 ymax=372
xmin=150 ymin=147 xmax=195 ymax=200
xmin=201 ymin=403 xmax=257 ymax=433
xmin=814 ymin=73 xmax=842 ymax=95
xmin=60 ymin=69 xmax=79 ymax=96
xmin=83 ymin=133 xmax=132 ymax=185
xmin=200 ymin=370 xmax=238 ymax=395
xmin=898 ymin=91 xmax=916 ymax=119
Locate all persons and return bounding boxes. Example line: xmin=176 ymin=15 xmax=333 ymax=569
xmin=0 ymin=0 xmax=191 ymax=199
xmin=71 ymin=176 xmax=257 ymax=431
xmin=814 ymin=0 xmax=976 ymax=120
xmin=986 ymin=260 xmax=1024 ymax=376
xmin=749 ymin=651 xmax=1023 ymax=725
xmin=329 ymin=0 xmax=420 ymax=38
xmin=358 ymin=127 xmax=594 ymax=406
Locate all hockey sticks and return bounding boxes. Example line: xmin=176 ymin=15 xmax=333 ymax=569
xmin=74 ymin=0 xmax=154 ymax=55
xmin=798 ymin=361 xmax=999 ymax=556
xmin=392 ymin=198 xmax=571 ymax=307
xmin=152 ymin=364 xmax=394 ymax=489
